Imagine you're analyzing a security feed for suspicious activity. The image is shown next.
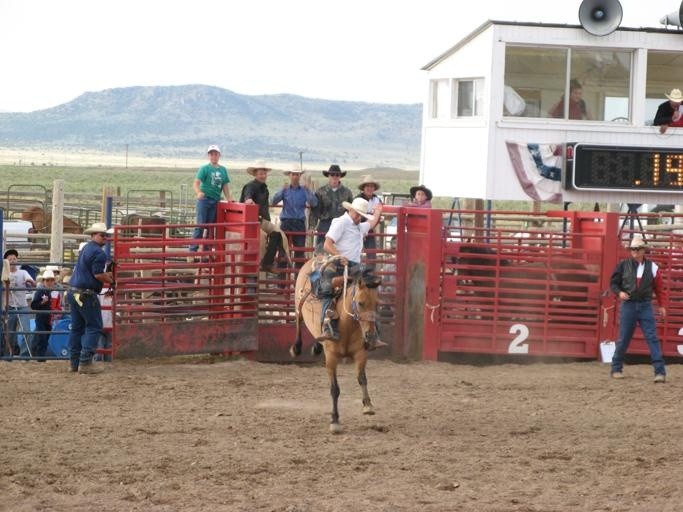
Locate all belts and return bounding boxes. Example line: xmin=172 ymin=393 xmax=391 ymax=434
xmin=67 ymin=286 xmax=96 ymax=296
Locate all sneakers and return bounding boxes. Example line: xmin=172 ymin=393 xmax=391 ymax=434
xmin=610 ymin=371 xmax=624 ymax=379
xmin=653 ymin=374 xmax=665 ymax=383
xmin=67 ymin=366 xmax=77 ymax=373
xmin=186 ymin=255 xmax=194 ymax=264
xmin=365 ymin=337 xmax=387 ymax=350
xmin=314 ymin=329 xmax=339 ymax=341
xmin=77 ymin=364 xmax=104 ymax=373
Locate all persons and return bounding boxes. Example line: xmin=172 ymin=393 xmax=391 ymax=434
xmin=188 ymin=145 xmax=233 ymax=263
xmin=354 ymin=174 xmax=386 ymax=272
xmin=654 ymin=89 xmax=683 ymax=134
xmin=271 ymin=162 xmax=319 ymax=294
xmin=409 ymin=185 xmax=431 ymax=208
xmin=1 ymin=221 xmax=115 ymax=374
xmin=238 ymin=162 xmax=284 ymax=274
xmin=609 ymin=237 xmax=669 ymax=382
xmin=308 ymin=164 xmax=353 ymax=257
xmin=552 ymin=80 xmax=586 ymax=119
xmin=309 ymin=196 xmax=390 ymax=348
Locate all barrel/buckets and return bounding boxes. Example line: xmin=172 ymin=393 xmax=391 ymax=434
xmin=46 ymin=319 xmax=73 ymax=360
xmin=600 ymin=339 xmax=615 ymax=363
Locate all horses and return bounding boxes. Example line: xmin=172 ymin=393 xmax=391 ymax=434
xmin=21 ymin=206 xmax=84 ymax=244
xmin=450 ymin=237 xmax=593 ymax=364
xmin=288 ymin=253 xmax=383 ymax=434
xmin=119 ymin=212 xmax=166 ymax=239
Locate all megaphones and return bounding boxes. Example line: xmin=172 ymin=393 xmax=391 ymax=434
xmin=659 ymin=0 xmax=683 ymax=30
xmin=578 ymin=0 xmax=623 ymax=36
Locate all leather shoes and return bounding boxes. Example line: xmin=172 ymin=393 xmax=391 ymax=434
xmin=263 ymin=264 xmax=281 ymax=275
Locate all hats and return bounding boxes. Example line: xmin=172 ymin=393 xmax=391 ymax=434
xmin=35 ymin=265 xmax=72 ymax=287
xmin=207 ymin=145 xmax=220 ymax=154
xmin=342 ymin=197 xmax=375 ymax=221
xmin=409 ymin=184 xmax=432 ymax=201
xmin=322 ymin=165 xmax=347 ymax=178
xmin=81 ymin=221 xmax=114 ymax=235
xmin=283 ymin=167 xmax=306 ymax=176
xmin=246 ymin=160 xmax=272 ymax=176
xmin=357 ymin=175 xmax=379 ymax=192
xmin=663 ymin=89 xmax=683 ymax=103
xmin=626 ymin=237 xmax=650 ymax=248
xmin=3 ymin=249 xmax=18 ymax=259
